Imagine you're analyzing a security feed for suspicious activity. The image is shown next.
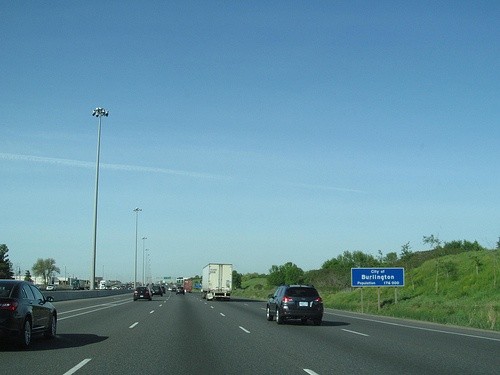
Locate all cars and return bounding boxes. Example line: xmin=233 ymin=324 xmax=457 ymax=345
xmin=105 ymin=285 xmax=112 ymax=290
xmin=176 ymin=287 xmax=185 ymax=295
xmin=133 ymin=286 xmax=153 ymax=301
xmin=112 ymin=285 xmax=127 ymax=290
xmin=161 ymin=286 xmax=166 ymax=294
xmin=169 ymin=287 xmax=172 ymax=290
xmin=46 ymin=284 xmax=57 ymax=290
xmin=150 ymin=285 xmax=164 ymax=297
xmin=171 ymin=287 xmax=176 ymax=291
xmin=266 ymin=284 xmax=324 ymax=325
xmin=0 ymin=279 xmax=58 ymax=345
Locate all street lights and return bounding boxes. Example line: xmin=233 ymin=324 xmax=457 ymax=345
xmin=89 ymin=107 xmax=109 ymax=290
xmin=142 ymin=237 xmax=151 ymax=285
xmin=132 ymin=207 xmax=143 ymax=290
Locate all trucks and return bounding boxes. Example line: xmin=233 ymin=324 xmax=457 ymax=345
xmin=100 ymin=281 xmax=107 ymax=290
xmin=200 ymin=263 xmax=233 ymax=301
xmin=182 ymin=280 xmax=192 ymax=293
